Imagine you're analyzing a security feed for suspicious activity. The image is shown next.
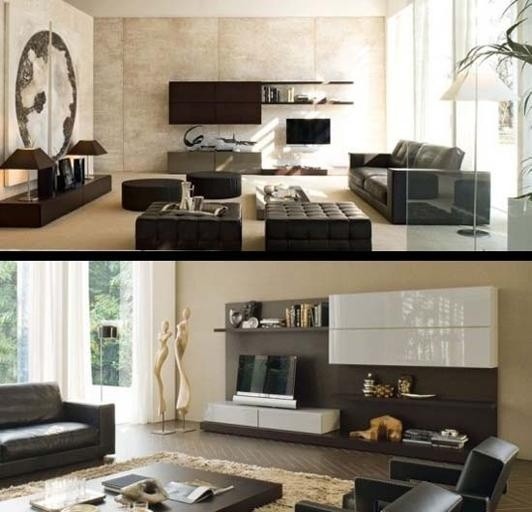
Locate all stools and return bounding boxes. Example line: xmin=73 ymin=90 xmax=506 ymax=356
xmin=296 ymin=437 xmax=519 ymax=510
xmin=134 ymin=198 xmax=372 ymax=255
xmin=122 ymin=171 xmax=241 ymax=212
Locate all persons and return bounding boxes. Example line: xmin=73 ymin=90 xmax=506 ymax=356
xmin=173 ymin=307 xmax=191 ymax=413
xmin=153 ymin=320 xmax=172 ymax=416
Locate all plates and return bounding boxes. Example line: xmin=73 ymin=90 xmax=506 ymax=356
xmin=30 ymin=486 xmax=107 ymax=512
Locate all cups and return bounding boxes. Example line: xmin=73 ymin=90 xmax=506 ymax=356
xmin=110 ymin=500 xmax=152 ymax=512
xmin=44 ymin=479 xmax=86 ymax=505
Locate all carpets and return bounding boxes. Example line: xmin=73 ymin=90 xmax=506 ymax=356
xmin=0 ymin=451 xmax=356 ymax=512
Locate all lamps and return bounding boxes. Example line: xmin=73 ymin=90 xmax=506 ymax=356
xmin=0 ymin=147 xmax=54 ymax=202
xmin=64 ymin=139 xmax=106 ymax=180
xmin=441 ymin=68 xmax=517 ymax=239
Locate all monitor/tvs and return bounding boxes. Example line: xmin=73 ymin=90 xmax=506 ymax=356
xmin=236 ymin=354 xmax=297 ymax=400
xmin=169 ymin=81 xmax=261 ymax=125
xmin=286 ymin=118 xmax=330 ymax=144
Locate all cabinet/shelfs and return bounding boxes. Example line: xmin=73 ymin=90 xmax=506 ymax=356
xmin=168 ymin=81 xmax=354 ymax=125
xmin=166 ymin=150 xmax=262 ymax=175
xmin=326 ymin=285 xmax=498 ymax=368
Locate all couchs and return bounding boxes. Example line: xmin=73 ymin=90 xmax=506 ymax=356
xmin=348 ymin=140 xmax=490 ymax=226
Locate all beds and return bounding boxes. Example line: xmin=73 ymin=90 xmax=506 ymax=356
xmin=0 ymin=381 xmax=115 ymax=478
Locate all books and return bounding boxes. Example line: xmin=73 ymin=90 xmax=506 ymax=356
xmin=160 ymin=477 xmax=234 ymax=505
xmin=260 ymin=85 xmax=309 ymax=103
xmin=101 ymin=472 xmax=154 ymax=494
xmin=259 ymin=299 xmax=328 ymax=328
xmin=401 ymin=428 xmax=469 ymax=450
